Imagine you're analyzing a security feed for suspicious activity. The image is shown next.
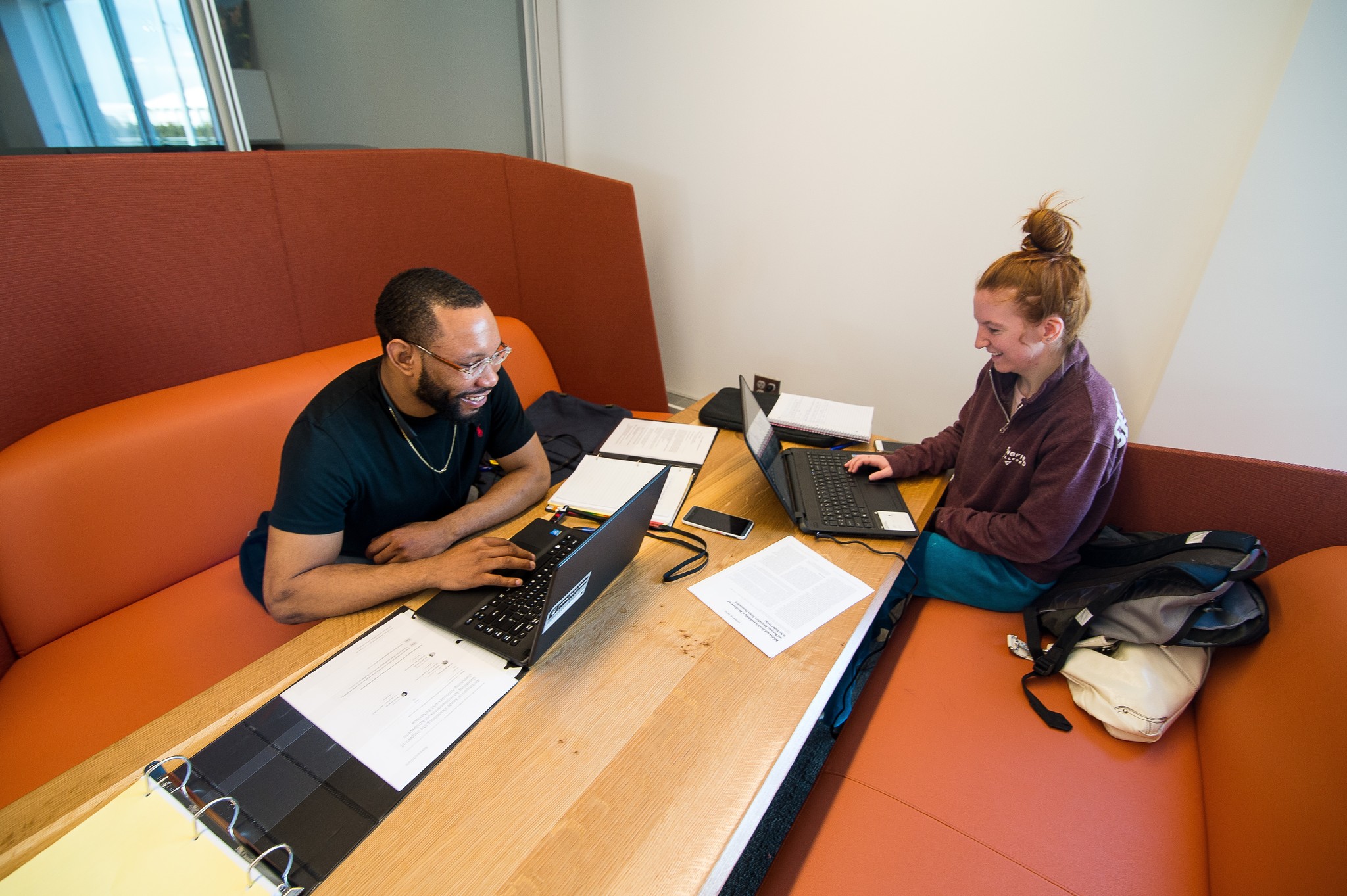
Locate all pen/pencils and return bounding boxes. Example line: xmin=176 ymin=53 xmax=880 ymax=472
xmin=830 ymin=441 xmax=862 ymax=450
xmin=572 ymin=526 xmax=596 ymax=533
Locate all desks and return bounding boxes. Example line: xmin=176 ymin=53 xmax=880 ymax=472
xmin=1 ymin=391 xmax=955 ymax=896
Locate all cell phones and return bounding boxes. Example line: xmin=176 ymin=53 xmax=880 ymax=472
xmin=682 ymin=506 xmax=754 ymax=540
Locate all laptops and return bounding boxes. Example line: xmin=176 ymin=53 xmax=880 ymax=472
xmin=414 ymin=464 xmax=673 ymax=667
xmin=739 ymin=375 xmax=920 ymax=540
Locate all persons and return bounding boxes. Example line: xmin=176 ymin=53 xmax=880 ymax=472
xmin=824 ymin=206 xmax=1128 ymax=730
xmin=260 ymin=267 xmax=551 ymax=624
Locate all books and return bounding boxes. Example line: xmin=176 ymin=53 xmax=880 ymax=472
xmin=765 ymin=393 xmax=877 ymax=444
xmin=544 ymin=416 xmax=721 ymax=529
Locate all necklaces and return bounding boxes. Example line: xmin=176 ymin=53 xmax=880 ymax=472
xmin=385 ymin=398 xmax=458 ymax=474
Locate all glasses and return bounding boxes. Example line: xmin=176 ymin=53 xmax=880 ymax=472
xmin=405 ymin=335 xmax=512 ymax=379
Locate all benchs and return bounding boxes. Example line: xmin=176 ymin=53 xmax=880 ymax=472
xmin=0 ymin=316 xmax=677 ymax=817
xmin=752 ymin=544 xmax=1347 ymax=896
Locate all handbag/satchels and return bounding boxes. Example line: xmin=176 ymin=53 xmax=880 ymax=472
xmin=1002 ymin=631 xmax=1211 ymax=744
xmin=474 ymin=391 xmax=632 ymax=507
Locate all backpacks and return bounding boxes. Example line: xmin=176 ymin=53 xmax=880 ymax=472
xmin=1022 ymin=525 xmax=1269 ymax=731
